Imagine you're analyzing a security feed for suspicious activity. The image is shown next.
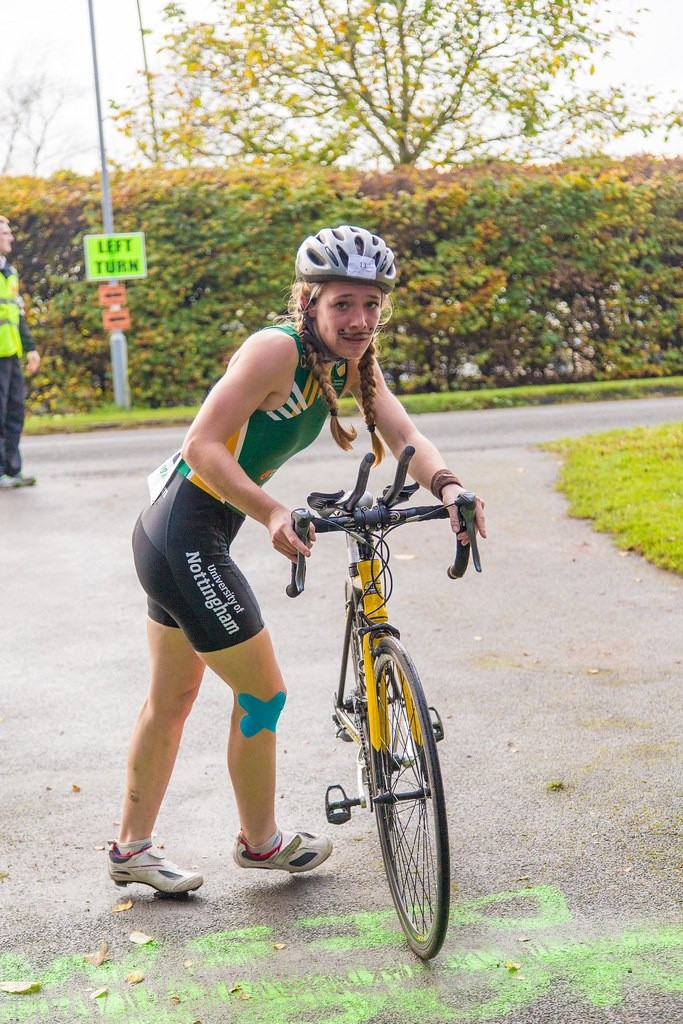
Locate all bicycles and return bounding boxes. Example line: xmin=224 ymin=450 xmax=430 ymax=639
xmin=286 ymin=445 xmax=482 ymax=961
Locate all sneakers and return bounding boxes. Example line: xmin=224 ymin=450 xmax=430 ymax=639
xmin=16 ymin=472 xmax=35 ymax=486
xmin=233 ymin=830 xmax=332 ymax=873
xmin=108 ymin=840 xmax=204 ymax=895
xmin=0 ymin=474 xmax=22 ymax=488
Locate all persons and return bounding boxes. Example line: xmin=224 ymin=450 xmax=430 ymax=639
xmin=107 ymin=225 xmax=486 ymax=894
xmin=0 ymin=215 xmax=40 ymax=488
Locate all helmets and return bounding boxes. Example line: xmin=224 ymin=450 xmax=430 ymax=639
xmin=294 ymin=225 xmax=397 ymax=294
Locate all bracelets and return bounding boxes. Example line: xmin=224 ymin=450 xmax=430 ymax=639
xmin=430 ymin=468 xmax=463 ymax=502
